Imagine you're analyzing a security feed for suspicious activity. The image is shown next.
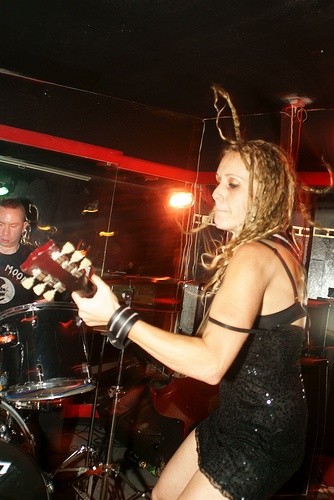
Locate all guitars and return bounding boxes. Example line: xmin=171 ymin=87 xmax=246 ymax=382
xmin=20 ymin=238 xmax=219 ymax=437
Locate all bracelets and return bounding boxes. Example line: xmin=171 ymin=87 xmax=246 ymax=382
xmin=101 ymin=305 xmax=140 ymax=351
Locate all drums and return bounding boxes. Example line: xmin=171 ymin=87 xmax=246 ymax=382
xmin=0 ymin=402 xmax=61 ymax=458
xmin=0 ymin=436 xmax=50 ymax=500
xmin=1 ymin=302 xmax=97 ymax=401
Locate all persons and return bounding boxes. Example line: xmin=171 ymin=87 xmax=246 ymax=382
xmin=70 ymin=139 xmax=312 ymax=500
xmin=0 ymin=197 xmax=55 ymax=314
